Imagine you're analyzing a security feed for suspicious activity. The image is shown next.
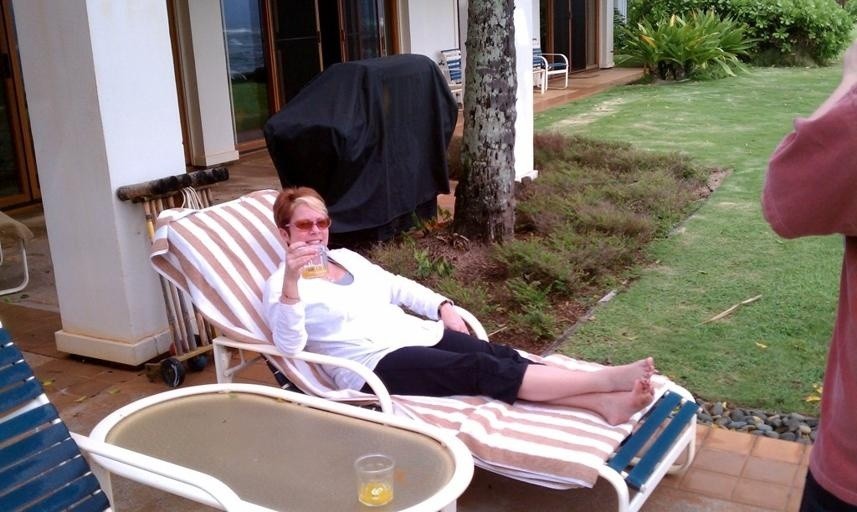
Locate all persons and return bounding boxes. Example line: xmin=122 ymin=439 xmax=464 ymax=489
xmin=261 ymin=184 xmax=659 ymax=427
xmin=762 ymin=40 xmax=856 ymax=512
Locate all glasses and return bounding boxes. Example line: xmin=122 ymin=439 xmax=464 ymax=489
xmin=287 ymin=213 xmax=333 ymax=234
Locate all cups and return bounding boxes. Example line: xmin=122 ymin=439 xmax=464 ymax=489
xmin=353 ymin=452 xmax=396 ymax=507
xmin=297 ymin=244 xmax=329 ymax=280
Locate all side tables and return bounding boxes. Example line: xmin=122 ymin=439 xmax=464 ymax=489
xmin=88 ymin=383 xmax=475 ymax=512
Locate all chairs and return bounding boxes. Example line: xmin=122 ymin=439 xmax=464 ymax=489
xmin=0 ymin=209 xmax=38 ymax=298
xmin=441 ymin=38 xmax=569 ymax=112
xmin=148 ymin=188 xmax=704 ymax=512
xmin=0 ymin=317 xmax=114 ymax=512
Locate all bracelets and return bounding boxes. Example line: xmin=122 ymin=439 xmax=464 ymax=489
xmin=437 ymin=300 xmax=454 ymax=317
xmin=282 ymin=290 xmax=300 ymax=301
